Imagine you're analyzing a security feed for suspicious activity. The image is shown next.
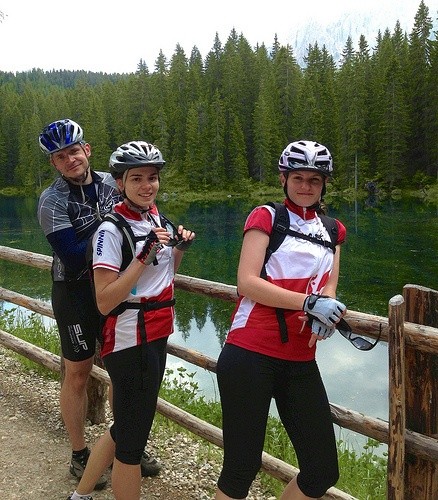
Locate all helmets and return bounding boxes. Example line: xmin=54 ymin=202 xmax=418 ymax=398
xmin=39 ymin=119 xmax=84 ymax=155
xmin=278 ymin=140 xmax=334 ymax=178
xmin=109 ymin=140 xmax=166 ymax=178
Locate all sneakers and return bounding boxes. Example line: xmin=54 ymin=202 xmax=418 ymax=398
xmin=140 ymin=453 xmax=160 ymax=474
xmin=69 ymin=452 xmax=107 ymax=490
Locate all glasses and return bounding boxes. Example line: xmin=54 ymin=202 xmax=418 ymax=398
xmin=336 ymin=302 xmax=381 ymax=350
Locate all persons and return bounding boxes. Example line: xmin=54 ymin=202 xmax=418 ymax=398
xmin=65 ymin=142 xmax=195 ymax=500
xmin=37 ymin=119 xmax=162 ymax=490
xmin=215 ymin=139 xmax=347 ymax=500
